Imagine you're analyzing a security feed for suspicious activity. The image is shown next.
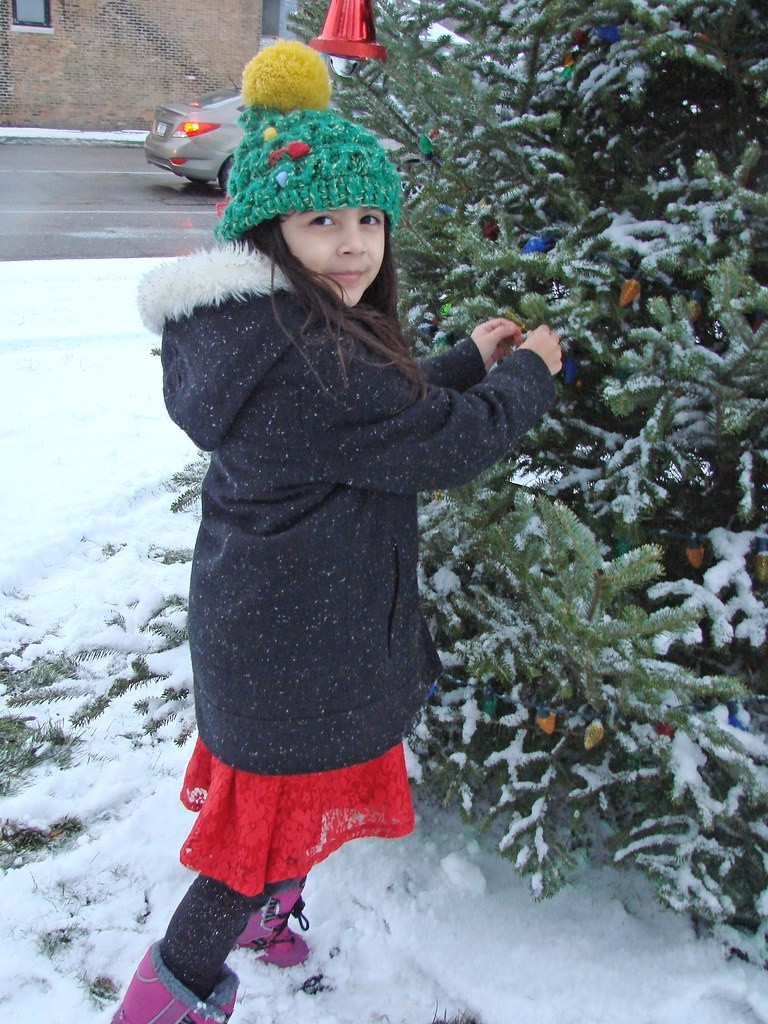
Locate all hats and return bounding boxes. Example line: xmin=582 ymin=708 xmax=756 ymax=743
xmin=215 ymin=37 xmax=403 ymax=242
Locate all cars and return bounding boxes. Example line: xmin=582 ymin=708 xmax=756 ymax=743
xmin=142 ymin=74 xmax=436 ymax=199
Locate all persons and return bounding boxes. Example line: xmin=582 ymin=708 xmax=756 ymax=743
xmin=111 ymin=40 xmax=562 ymax=1024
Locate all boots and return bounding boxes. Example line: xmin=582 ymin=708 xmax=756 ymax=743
xmin=109 ymin=938 xmax=240 ymax=1024
xmin=234 ymin=874 xmax=310 ymax=967
